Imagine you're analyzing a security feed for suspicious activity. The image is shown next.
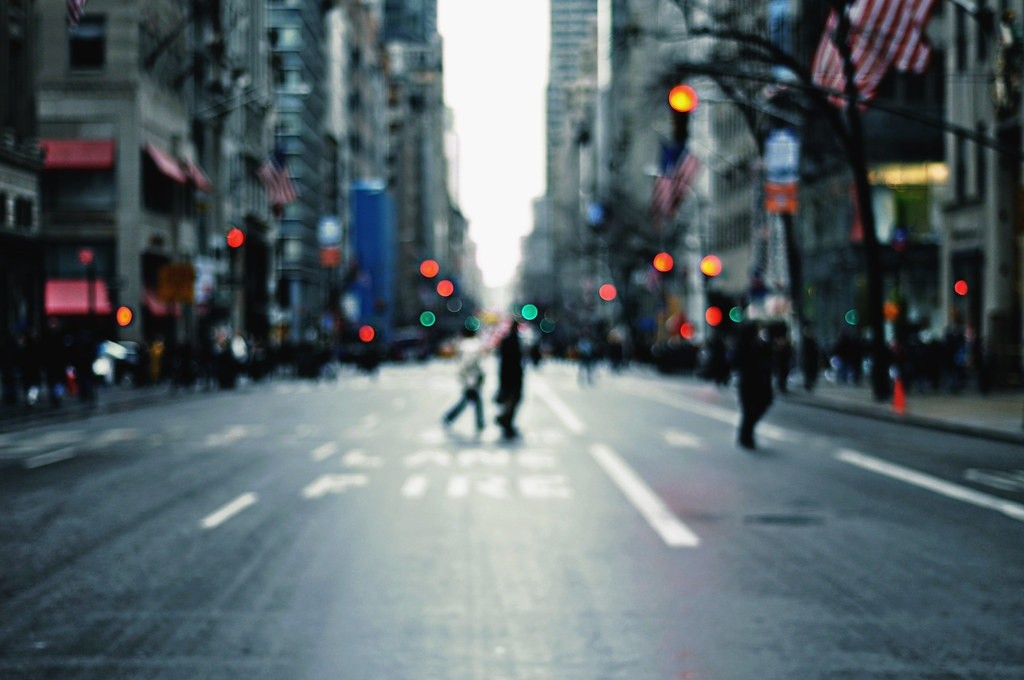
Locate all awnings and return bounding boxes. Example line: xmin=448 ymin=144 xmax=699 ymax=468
xmin=144 ymin=141 xmax=213 ymax=191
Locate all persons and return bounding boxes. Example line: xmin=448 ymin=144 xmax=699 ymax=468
xmin=492 ymin=320 xmax=524 ymax=440
xmin=530 ymin=312 xmax=970 ymax=450
xmin=438 ymin=325 xmax=486 ymax=433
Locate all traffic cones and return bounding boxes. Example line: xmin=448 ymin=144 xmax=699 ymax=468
xmin=893 ymin=376 xmax=911 ymax=412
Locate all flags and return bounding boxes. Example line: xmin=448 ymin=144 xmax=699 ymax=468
xmin=811 ymin=0 xmax=935 ymax=112
xmin=650 ymin=134 xmax=698 ymax=223
xmin=255 ymin=149 xmax=299 ymax=211
